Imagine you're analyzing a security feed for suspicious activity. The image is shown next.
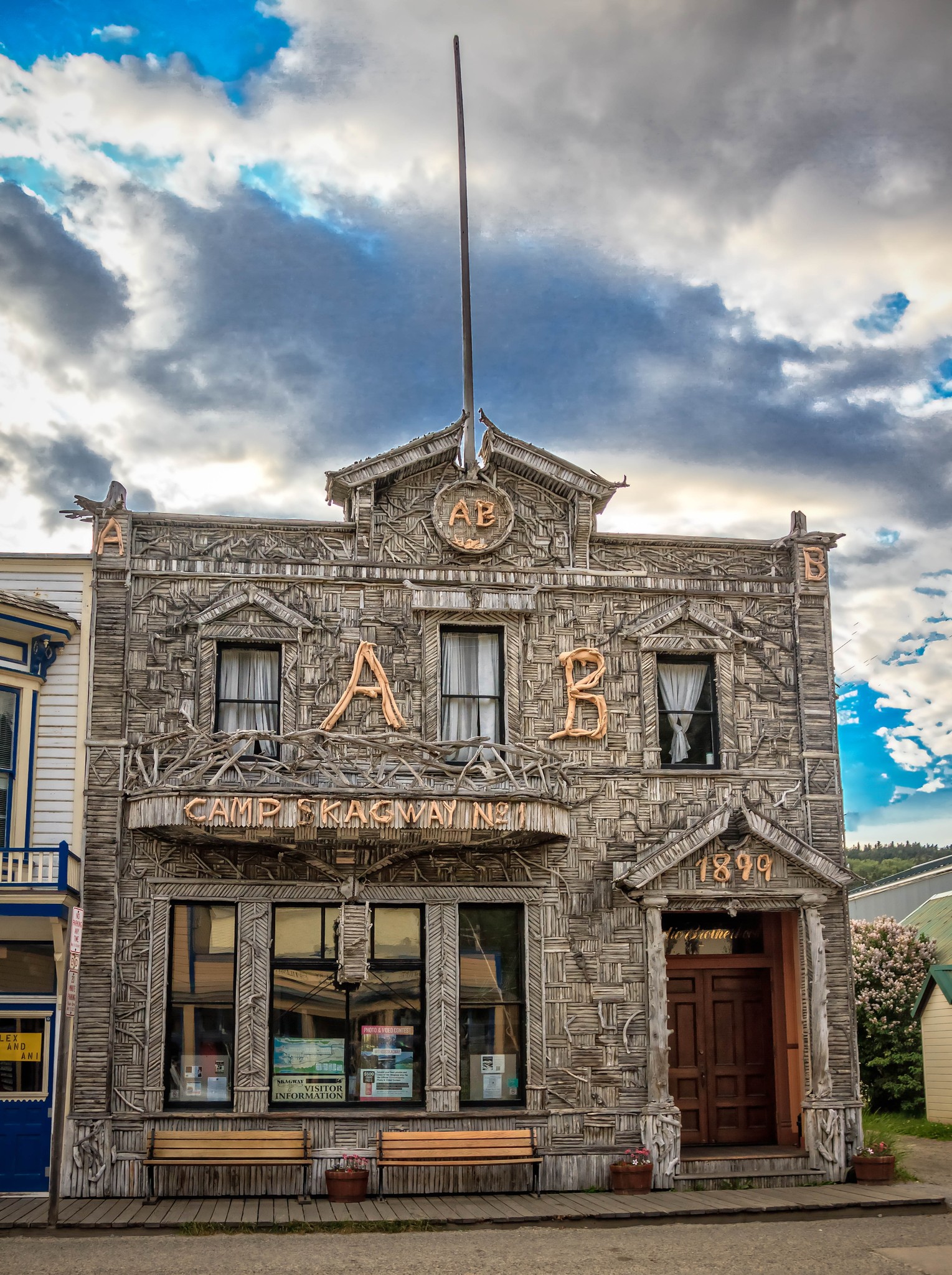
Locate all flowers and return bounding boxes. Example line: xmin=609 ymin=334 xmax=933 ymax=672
xmin=330 ymin=1154 xmax=368 ymax=1173
xmin=614 ymin=1147 xmax=650 ymax=1166
xmin=854 ymin=1142 xmax=888 ymax=1158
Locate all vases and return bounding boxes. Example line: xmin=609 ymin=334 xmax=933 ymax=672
xmin=325 ymin=1168 xmax=369 ymax=1203
xmin=610 ymin=1162 xmax=653 ymax=1195
xmin=853 ymin=1155 xmax=895 ymax=1186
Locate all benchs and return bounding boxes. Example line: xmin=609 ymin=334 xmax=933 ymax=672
xmin=375 ymin=1131 xmax=543 ymax=1202
xmin=140 ymin=1128 xmax=312 ymax=1205
